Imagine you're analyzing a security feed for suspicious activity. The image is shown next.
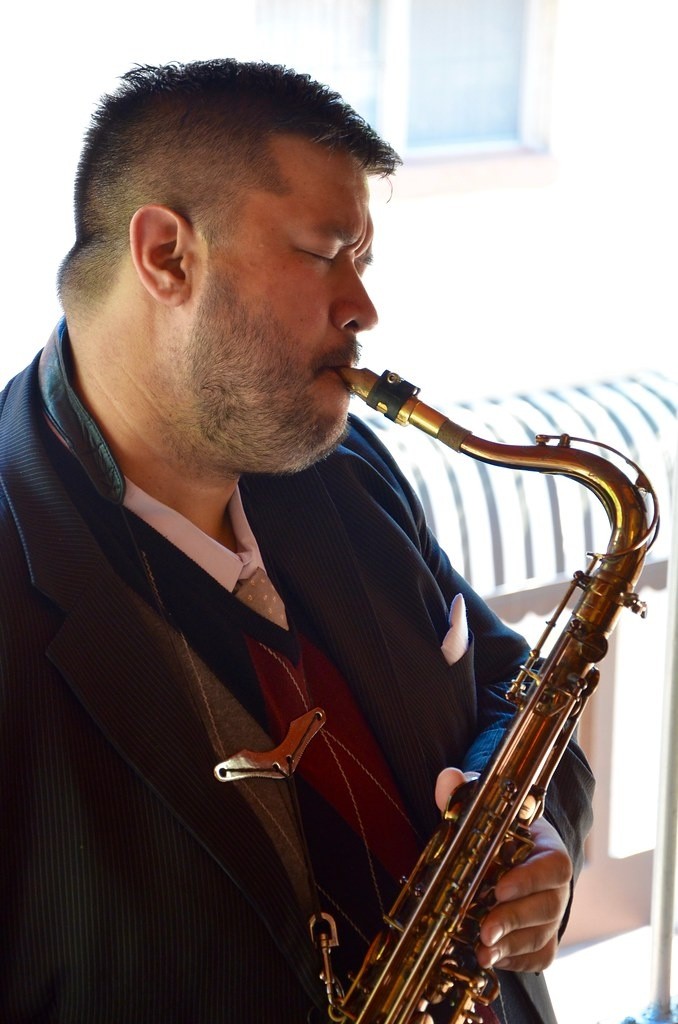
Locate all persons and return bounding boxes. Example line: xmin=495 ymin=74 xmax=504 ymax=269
xmin=0 ymin=57 xmax=594 ymax=1024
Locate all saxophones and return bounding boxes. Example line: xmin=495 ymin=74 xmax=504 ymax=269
xmin=306 ymin=363 xmax=661 ymax=1024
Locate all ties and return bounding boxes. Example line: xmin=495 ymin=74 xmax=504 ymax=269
xmin=234 ymin=567 xmax=290 ymax=631
